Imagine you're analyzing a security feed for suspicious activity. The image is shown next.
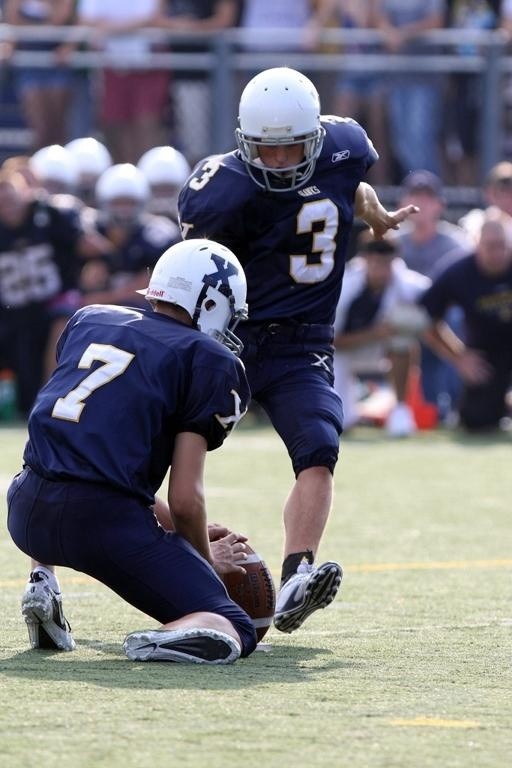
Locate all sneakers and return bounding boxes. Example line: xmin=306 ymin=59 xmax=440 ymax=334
xmin=392 ymin=406 xmax=416 ymax=436
xmin=274 ymin=561 xmax=342 ymax=633
xmin=21 ymin=572 xmax=77 ymax=650
xmin=123 ymin=628 xmax=241 ymax=665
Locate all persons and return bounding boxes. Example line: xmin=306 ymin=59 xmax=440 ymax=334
xmin=174 ymin=61 xmax=420 ymax=632
xmin=0 ymin=235 xmax=261 ymax=668
xmin=2 ymin=1 xmax=511 ymax=437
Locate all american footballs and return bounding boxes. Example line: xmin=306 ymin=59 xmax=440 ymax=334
xmin=204 ymin=524 xmax=276 ymax=643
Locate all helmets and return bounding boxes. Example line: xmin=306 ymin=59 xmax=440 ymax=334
xmin=28 ymin=137 xmax=190 ymax=202
xmin=234 ymin=65 xmax=327 ymax=194
xmin=146 ymin=237 xmax=251 ymax=357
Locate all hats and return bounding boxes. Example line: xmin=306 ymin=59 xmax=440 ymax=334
xmin=405 ymin=169 xmax=443 ymax=198
